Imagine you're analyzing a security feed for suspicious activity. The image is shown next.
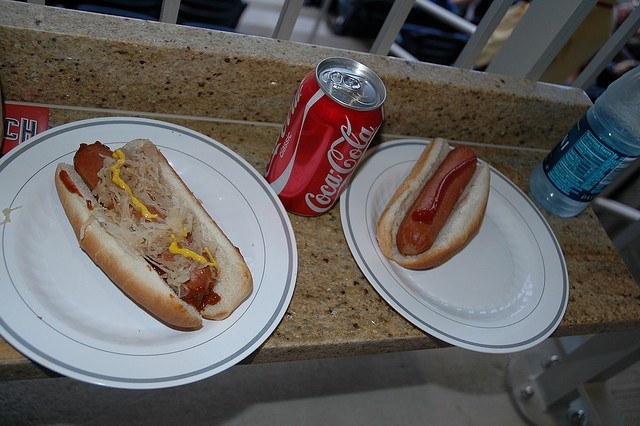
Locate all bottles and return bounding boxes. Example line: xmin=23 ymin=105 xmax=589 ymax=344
xmin=528 ymin=65 xmax=640 ymax=220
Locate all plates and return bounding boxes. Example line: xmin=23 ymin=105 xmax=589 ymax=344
xmin=0 ymin=115 xmax=299 ymax=390
xmin=339 ymin=138 xmax=570 ymax=354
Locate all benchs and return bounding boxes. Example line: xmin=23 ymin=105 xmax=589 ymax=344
xmin=2 ymin=0 xmax=639 ymax=378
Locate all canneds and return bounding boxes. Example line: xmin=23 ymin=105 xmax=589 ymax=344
xmin=260 ymin=56 xmax=388 ymax=218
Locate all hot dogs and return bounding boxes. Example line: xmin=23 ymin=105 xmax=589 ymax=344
xmin=374 ymin=138 xmax=491 ymax=272
xmin=51 ymin=138 xmax=255 ymax=331
